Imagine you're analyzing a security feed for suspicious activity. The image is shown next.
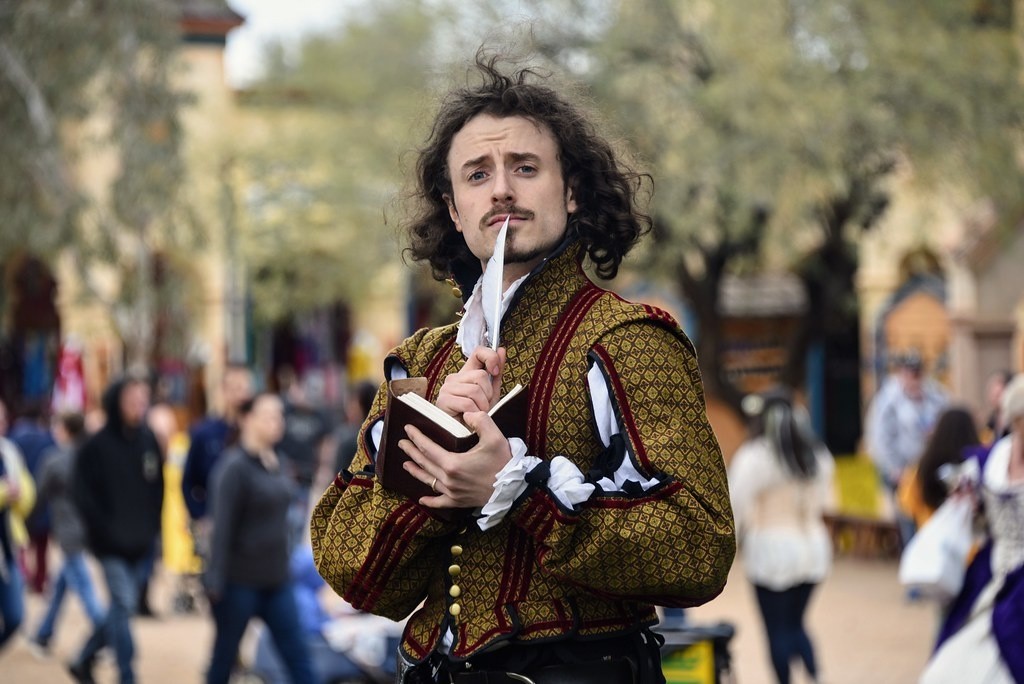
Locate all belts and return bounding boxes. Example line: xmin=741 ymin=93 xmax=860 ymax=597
xmin=434 ymin=669 xmax=510 ymax=684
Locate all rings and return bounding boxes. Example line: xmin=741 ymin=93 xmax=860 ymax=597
xmin=429 ymin=478 xmax=437 ymax=492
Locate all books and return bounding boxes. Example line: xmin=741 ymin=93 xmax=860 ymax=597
xmin=375 ymin=376 xmax=531 ymax=531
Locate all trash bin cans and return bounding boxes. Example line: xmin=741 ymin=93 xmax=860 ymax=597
xmin=646 ymin=621 xmax=734 ymax=684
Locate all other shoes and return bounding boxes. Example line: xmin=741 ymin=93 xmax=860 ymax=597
xmin=69 ymin=661 xmax=95 ymax=683
xmin=25 ymin=636 xmax=51 ymax=661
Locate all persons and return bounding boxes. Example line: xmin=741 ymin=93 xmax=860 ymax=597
xmin=725 ymin=383 xmax=844 ymax=684
xmin=776 ymin=147 xmax=1024 ymax=684
xmin=311 ymin=26 xmax=738 ymax=683
xmin=1 ymin=371 xmax=377 ymax=683
